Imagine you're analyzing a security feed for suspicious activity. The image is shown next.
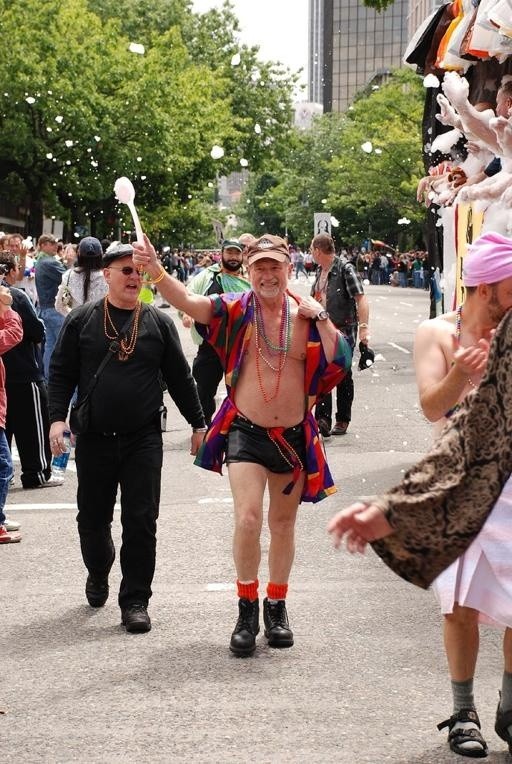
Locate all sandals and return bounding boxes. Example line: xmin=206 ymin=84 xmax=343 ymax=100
xmin=447 ymin=708 xmax=491 ymax=759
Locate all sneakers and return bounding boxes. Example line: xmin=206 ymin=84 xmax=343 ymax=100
xmin=40 ymin=469 xmax=66 ymax=487
xmin=316 ymin=416 xmax=332 ymax=439
xmin=1 ymin=517 xmax=23 ymax=544
xmin=494 ymin=700 xmax=511 ymax=751
xmin=120 ymin=605 xmax=152 ymax=633
xmin=331 ymin=419 xmax=349 ymax=435
xmin=84 ymin=571 xmax=109 ymax=607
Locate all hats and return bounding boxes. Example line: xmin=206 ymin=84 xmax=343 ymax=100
xmin=99 ymin=241 xmax=134 ymax=267
xmin=247 ymin=234 xmax=290 ymax=268
xmin=222 ymin=237 xmax=245 ymax=254
xmin=38 ymin=234 xmax=56 ymax=245
xmin=80 ymin=236 xmax=104 ymax=259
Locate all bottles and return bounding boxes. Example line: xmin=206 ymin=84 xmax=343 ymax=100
xmin=50 ymin=429 xmax=72 ymax=485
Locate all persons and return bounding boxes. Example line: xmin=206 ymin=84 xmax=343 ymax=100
xmin=131 ymin=233 xmax=353 ymax=655
xmin=412 ymin=232 xmax=512 ymax=763
xmin=46 ymin=244 xmax=207 ymax=632
xmin=1 ymin=231 xmax=512 ymax=588
xmin=413 ymin=70 xmax=511 ymax=210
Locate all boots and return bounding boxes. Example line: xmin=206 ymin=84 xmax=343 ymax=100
xmin=263 ymin=596 xmax=294 ymax=648
xmin=228 ymin=596 xmax=262 ymax=658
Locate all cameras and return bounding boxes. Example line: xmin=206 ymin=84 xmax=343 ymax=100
xmin=0 ymin=264 xmax=9 ymax=276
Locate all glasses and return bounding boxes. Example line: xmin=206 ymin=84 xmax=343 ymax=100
xmin=110 ymin=266 xmax=143 ymax=276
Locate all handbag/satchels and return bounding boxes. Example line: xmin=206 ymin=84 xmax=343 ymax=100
xmin=60 ymin=270 xmax=74 ymax=315
xmin=68 ymin=394 xmax=91 ymax=435
